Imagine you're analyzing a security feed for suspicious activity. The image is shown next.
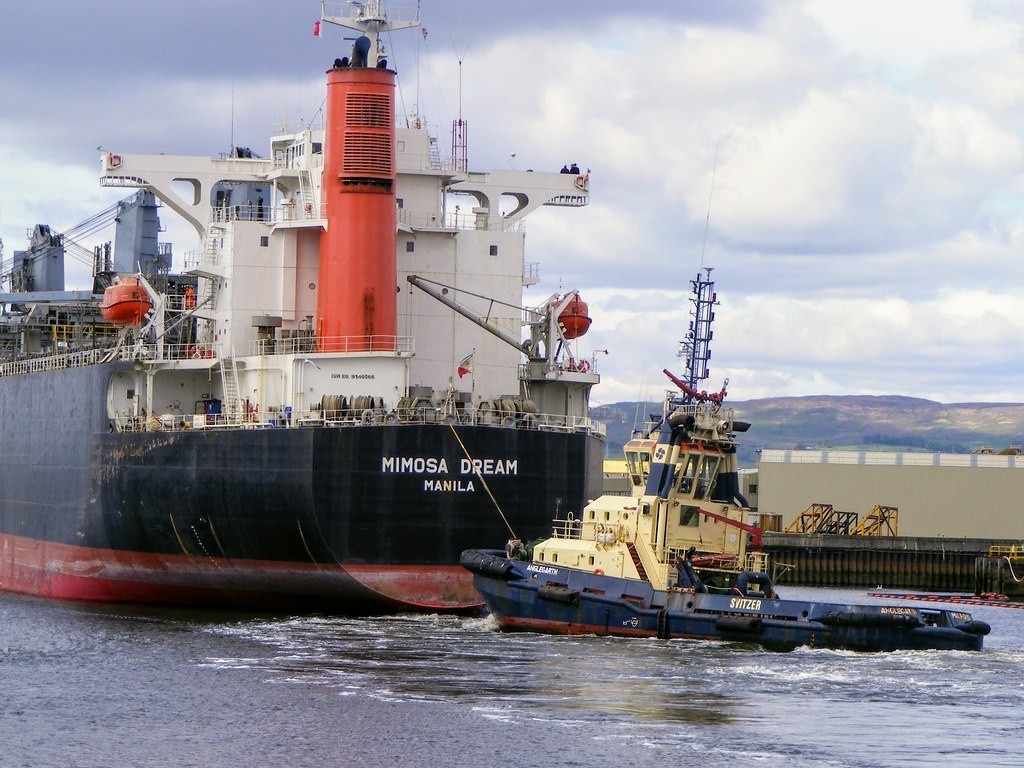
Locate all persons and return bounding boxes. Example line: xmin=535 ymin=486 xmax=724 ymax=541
xmin=560 ymin=163 xmax=581 ymax=174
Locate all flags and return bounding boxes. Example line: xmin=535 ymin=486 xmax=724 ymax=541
xmin=422 ymin=28 xmax=428 ymax=40
xmin=314 ymin=21 xmax=320 ymax=36
xmin=458 ymin=353 xmax=474 ymax=379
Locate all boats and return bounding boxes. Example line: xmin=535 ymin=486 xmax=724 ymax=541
xmin=1 ymin=1 xmax=995 ymax=689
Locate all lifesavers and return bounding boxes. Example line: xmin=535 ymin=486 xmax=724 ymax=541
xmin=385 ymin=413 xmax=398 ymax=425
xmin=444 ymin=414 xmax=457 ymax=425
xmin=501 ymin=416 xmax=515 ymax=428
xmin=591 ymin=565 xmax=607 ymax=578
xmin=576 ymin=357 xmax=591 ymax=374
xmin=191 ymin=345 xmax=205 ymax=359
